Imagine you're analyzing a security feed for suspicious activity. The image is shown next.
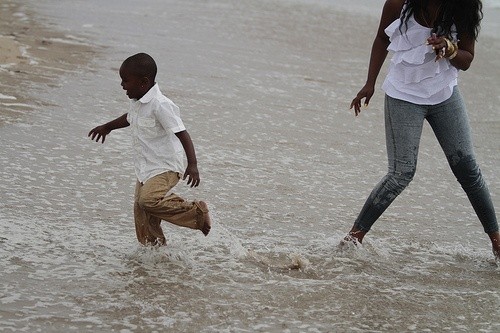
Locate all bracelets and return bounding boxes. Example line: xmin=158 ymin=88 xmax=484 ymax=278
xmin=444 ymin=37 xmax=454 ymax=59
xmin=449 ymin=42 xmax=459 ymax=60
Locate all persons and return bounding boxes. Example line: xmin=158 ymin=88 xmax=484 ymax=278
xmin=87 ymin=53 xmax=211 ymax=248
xmin=339 ymin=0 xmax=499 ymax=265
